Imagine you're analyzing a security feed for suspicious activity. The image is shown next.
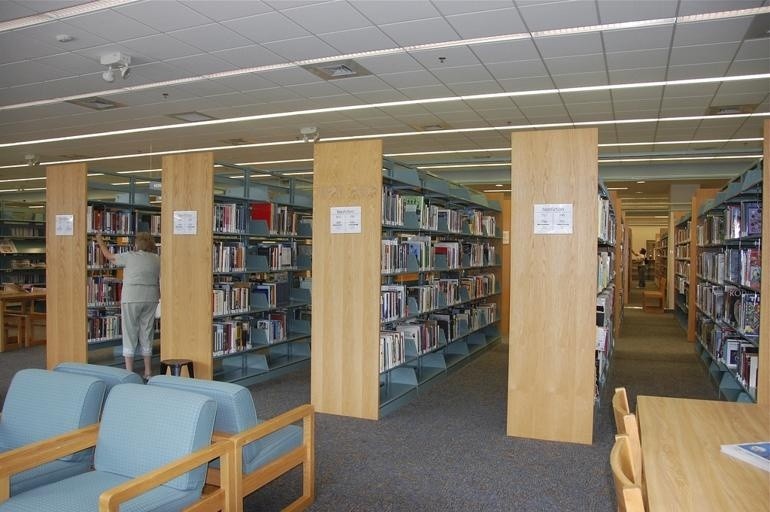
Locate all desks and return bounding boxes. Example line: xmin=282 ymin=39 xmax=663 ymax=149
xmin=638 ymin=393 xmax=770 ymax=511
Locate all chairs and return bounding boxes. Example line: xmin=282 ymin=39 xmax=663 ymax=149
xmin=608 ymin=383 xmax=643 ymax=482
xmin=2 ymin=367 xmax=109 ymax=497
xmin=603 ymin=432 xmax=648 ymax=512
xmin=148 ymin=374 xmax=316 ymax=511
xmin=1 ymin=383 xmax=236 ymax=512
xmin=52 ymin=360 xmax=145 ymax=422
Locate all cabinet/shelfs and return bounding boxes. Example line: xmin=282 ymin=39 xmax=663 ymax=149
xmin=44 ymin=162 xmax=161 ymax=372
xmin=645 ymin=195 xmax=696 ymax=313
xmin=309 ymin=138 xmax=503 ymax=422
xmin=506 ymin=128 xmax=630 ymax=449
xmin=159 ymin=151 xmax=312 ymax=387
xmin=1 ymin=199 xmax=47 ymax=353
xmin=691 ymin=119 xmax=768 ymax=410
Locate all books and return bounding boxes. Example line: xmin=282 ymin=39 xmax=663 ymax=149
xmin=719 ymin=441 xmax=770 ymax=473
xmin=596 ymin=192 xmax=625 ymax=402
xmin=654 ymin=204 xmax=762 ymax=402
xmin=3 ymin=227 xmax=40 ymax=283
xmin=87 ymin=206 xmax=161 ymax=344
xmin=212 ymin=203 xmax=297 ymax=359
xmin=379 ymin=185 xmax=498 ymax=373
